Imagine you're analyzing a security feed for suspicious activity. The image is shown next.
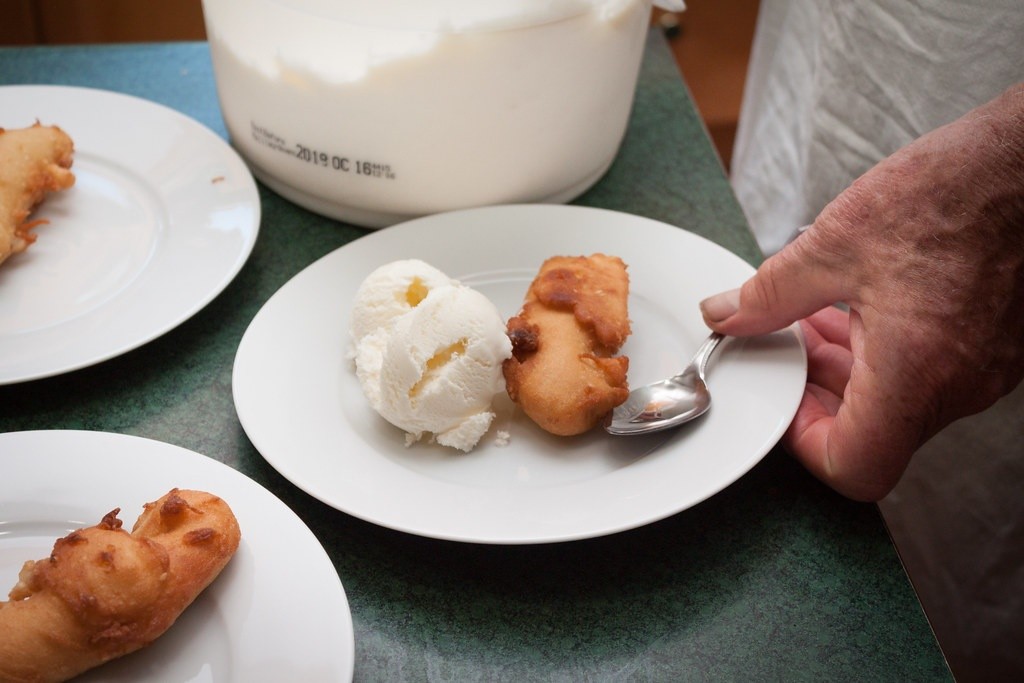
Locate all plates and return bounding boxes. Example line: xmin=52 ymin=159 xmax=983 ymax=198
xmin=0 ymin=429 xmax=355 ymax=683
xmin=0 ymin=82 xmax=260 ymax=380
xmin=230 ymin=201 xmax=810 ymax=545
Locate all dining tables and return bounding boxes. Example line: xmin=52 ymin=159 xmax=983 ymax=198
xmin=0 ymin=10 xmax=960 ymax=683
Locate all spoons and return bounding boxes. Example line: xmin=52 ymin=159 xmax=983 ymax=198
xmin=602 ymin=332 xmax=726 ymax=436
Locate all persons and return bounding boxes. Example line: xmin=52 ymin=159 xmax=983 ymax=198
xmin=699 ymin=0 xmax=1023 ymax=683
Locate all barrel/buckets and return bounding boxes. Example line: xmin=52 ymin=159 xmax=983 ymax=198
xmin=197 ymin=1 xmax=688 ymax=227
